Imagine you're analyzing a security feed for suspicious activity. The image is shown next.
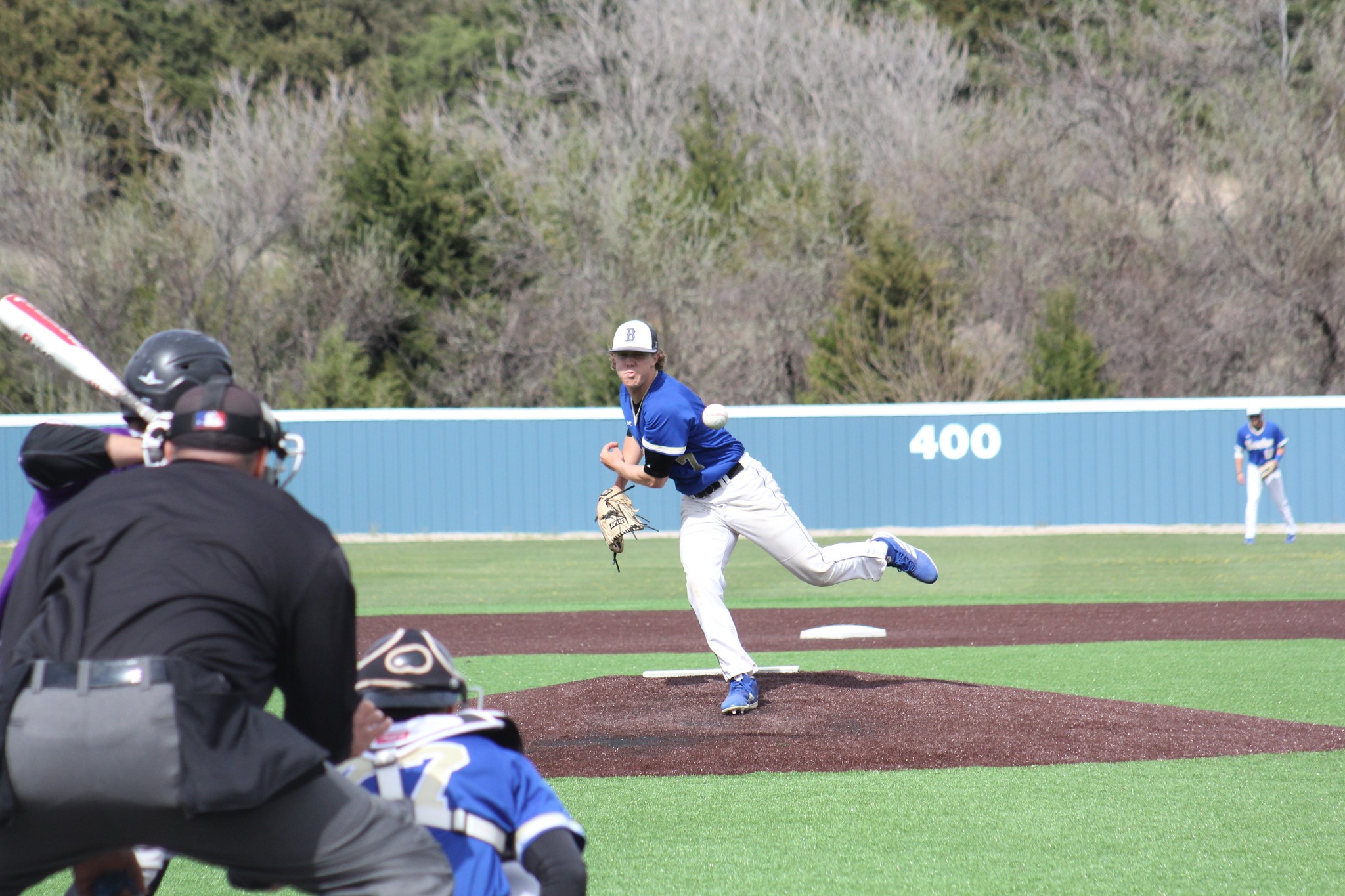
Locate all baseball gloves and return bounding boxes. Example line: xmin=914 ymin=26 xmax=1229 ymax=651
xmin=594 ymin=485 xmax=660 ymax=574
xmin=1258 ymin=460 xmax=1277 ymax=479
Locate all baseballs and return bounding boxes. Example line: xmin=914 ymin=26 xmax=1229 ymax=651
xmin=702 ymin=403 xmax=728 ymax=430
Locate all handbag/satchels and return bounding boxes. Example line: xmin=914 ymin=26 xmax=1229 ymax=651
xmin=174 ymin=672 xmax=331 ymax=815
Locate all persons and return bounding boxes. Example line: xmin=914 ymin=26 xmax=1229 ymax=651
xmin=1234 ymin=404 xmax=1299 ymax=544
xmin=0 ymin=328 xmax=454 ymax=894
xmin=595 ymin=318 xmax=940 ymax=715
xmin=226 ymin=628 xmax=592 ymax=896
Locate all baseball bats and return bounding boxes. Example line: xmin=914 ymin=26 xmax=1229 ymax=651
xmin=3 ymin=293 xmax=161 ymax=425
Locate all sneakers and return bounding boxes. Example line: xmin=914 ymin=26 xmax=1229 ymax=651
xmin=721 ymin=671 xmax=759 ymax=714
xmin=870 ymin=531 xmax=938 ymax=585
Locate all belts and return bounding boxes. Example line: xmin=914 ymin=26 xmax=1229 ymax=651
xmin=27 ymin=658 xmax=171 ymax=688
xmin=687 ymin=461 xmax=744 ymax=499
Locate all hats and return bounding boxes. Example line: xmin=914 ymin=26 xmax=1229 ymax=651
xmin=609 ymin=320 xmax=660 ymax=355
xmin=1246 ymin=404 xmax=1265 ymax=415
xmin=168 ymin=383 xmax=264 ymax=451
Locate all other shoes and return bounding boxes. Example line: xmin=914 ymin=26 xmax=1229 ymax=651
xmin=1285 ymin=535 xmax=1296 ymax=543
xmin=1243 ymin=538 xmax=1254 ymax=545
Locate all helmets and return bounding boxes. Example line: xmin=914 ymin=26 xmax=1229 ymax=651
xmin=121 ymin=328 xmax=237 ymax=419
xmin=354 ymin=624 xmax=468 ymax=712
